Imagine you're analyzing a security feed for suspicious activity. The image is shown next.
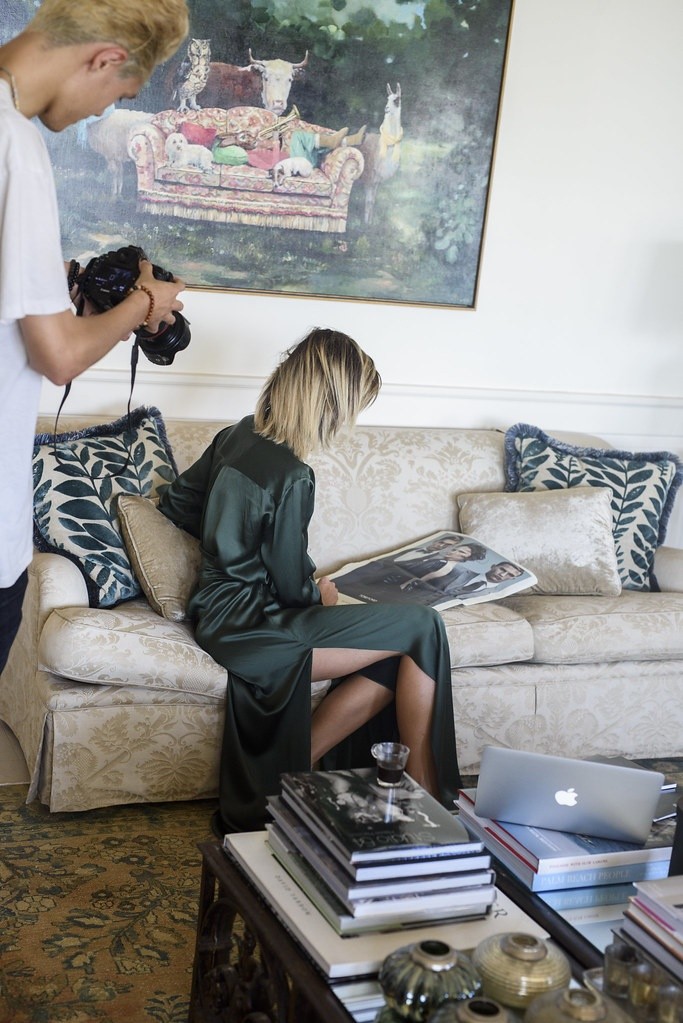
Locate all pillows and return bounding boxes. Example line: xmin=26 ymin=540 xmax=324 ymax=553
xmin=504 ymin=423 xmax=683 ymax=592
xmin=33 ymin=406 xmax=180 ymax=609
xmin=458 ymin=487 xmax=622 ymax=596
xmin=117 ymin=495 xmax=202 ymax=622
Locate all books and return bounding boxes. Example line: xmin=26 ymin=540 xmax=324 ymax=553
xmin=222 ymin=767 xmax=550 ymax=1023
xmin=609 ymin=872 xmax=683 ymax=985
xmin=453 ymin=772 xmax=679 ymax=892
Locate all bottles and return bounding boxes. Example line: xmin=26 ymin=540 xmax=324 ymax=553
xmin=376 ymin=931 xmax=633 ymax=1023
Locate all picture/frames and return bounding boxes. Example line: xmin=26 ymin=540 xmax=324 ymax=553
xmin=1 ymin=0 xmax=516 ymax=311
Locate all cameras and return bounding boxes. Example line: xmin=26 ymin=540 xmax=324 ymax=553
xmin=80 ymin=244 xmax=192 ymax=366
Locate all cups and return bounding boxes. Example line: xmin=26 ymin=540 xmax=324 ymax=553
xmin=602 ymin=943 xmax=683 ymax=1023
xmin=373 ymin=742 xmax=411 ymax=787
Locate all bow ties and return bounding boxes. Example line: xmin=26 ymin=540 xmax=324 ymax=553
xmin=416 ymin=549 xmax=432 ymax=554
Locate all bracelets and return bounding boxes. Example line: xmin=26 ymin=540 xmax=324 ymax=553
xmin=127 ymin=285 xmax=155 ymax=326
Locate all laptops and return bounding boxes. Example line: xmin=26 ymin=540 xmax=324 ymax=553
xmin=474 ymin=745 xmax=665 ymax=846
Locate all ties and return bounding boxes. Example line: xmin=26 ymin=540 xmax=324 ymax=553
xmin=462 ymin=581 xmax=487 ymax=591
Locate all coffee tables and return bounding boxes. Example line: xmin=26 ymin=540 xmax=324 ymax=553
xmin=190 ymin=810 xmax=682 ymax=1023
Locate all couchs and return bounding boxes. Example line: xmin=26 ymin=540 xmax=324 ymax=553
xmin=1 ymin=412 xmax=683 ymax=814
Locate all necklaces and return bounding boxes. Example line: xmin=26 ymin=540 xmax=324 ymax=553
xmin=0 ymin=67 xmax=20 ymax=110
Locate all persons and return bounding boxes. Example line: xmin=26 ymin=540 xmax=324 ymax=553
xmin=0 ymin=0 xmax=191 ymax=674
xmin=330 ymin=535 xmax=524 ymax=607
xmin=156 ymin=325 xmax=466 ymax=841
